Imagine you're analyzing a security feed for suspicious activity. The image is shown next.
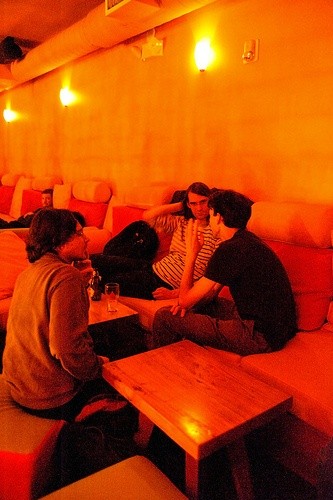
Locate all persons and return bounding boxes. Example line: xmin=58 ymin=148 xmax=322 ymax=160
xmin=89 ymin=182 xmax=223 ymax=357
xmin=0 ymin=189 xmax=53 ymax=229
xmin=153 ymin=190 xmax=297 ymax=357
xmin=2 ymin=207 xmax=157 ymax=464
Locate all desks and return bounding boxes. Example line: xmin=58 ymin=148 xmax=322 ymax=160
xmin=84 ymin=288 xmax=138 ymax=353
xmin=101 ymin=338 xmax=292 ymax=500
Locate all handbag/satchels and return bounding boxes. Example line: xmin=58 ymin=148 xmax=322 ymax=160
xmin=60 ymin=426 xmax=136 ymax=485
xmin=103 ymin=220 xmax=158 ymax=261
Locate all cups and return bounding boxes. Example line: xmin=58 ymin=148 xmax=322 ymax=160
xmin=104 ymin=282 xmax=120 ymax=312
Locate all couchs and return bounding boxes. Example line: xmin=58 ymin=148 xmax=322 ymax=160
xmin=0 ymin=175 xmax=333 ymax=500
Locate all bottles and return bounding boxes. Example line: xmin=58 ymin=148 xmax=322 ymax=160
xmin=90 ymin=266 xmax=101 ymax=301
